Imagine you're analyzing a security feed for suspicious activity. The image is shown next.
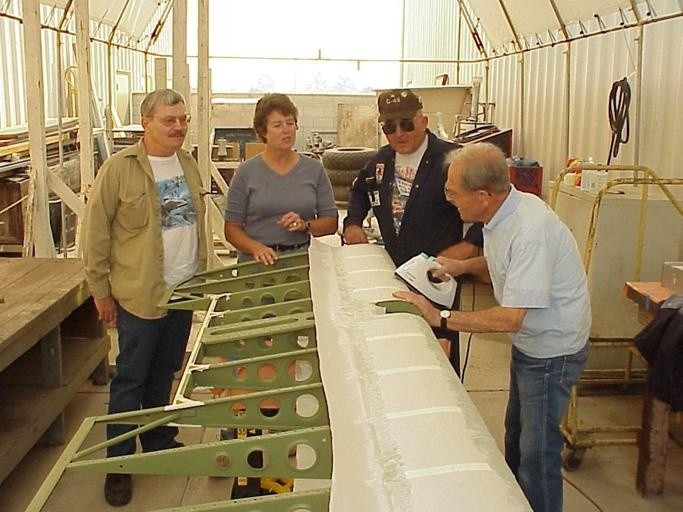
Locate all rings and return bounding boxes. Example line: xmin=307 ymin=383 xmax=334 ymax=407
xmin=293 ymin=222 xmax=297 ymax=228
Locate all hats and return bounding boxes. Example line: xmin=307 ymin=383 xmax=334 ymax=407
xmin=377 ymin=88 xmax=424 ymax=122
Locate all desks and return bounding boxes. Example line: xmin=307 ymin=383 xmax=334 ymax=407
xmin=1 ymin=256 xmax=112 ymax=489
xmin=623 ymin=280 xmax=681 ymax=498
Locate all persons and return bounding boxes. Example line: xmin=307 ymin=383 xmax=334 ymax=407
xmin=77 ymin=89 xmax=206 ymax=507
xmin=224 ymin=94 xmax=339 ymax=268
xmin=392 ymin=141 xmax=591 ymax=512
xmin=343 ymin=89 xmax=485 ymax=377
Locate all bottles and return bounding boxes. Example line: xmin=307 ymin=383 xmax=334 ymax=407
xmin=579 ymin=156 xmax=608 ymax=198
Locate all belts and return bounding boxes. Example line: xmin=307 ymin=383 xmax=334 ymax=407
xmin=266 ymin=239 xmax=310 ymax=252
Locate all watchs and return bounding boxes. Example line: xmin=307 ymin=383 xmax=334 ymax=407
xmin=440 ymin=309 xmax=451 ymax=329
xmin=302 ymin=218 xmax=311 ymax=232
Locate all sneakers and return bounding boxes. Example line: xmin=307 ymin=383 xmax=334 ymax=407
xmin=142 ymin=438 xmax=185 ymax=453
xmin=104 ymin=473 xmax=132 ymax=507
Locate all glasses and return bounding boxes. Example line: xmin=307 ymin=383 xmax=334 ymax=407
xmin=147 ymin=113 xmax=192 ymax=127
xmin=382 ymin=114 xmax=421 ymax=135
